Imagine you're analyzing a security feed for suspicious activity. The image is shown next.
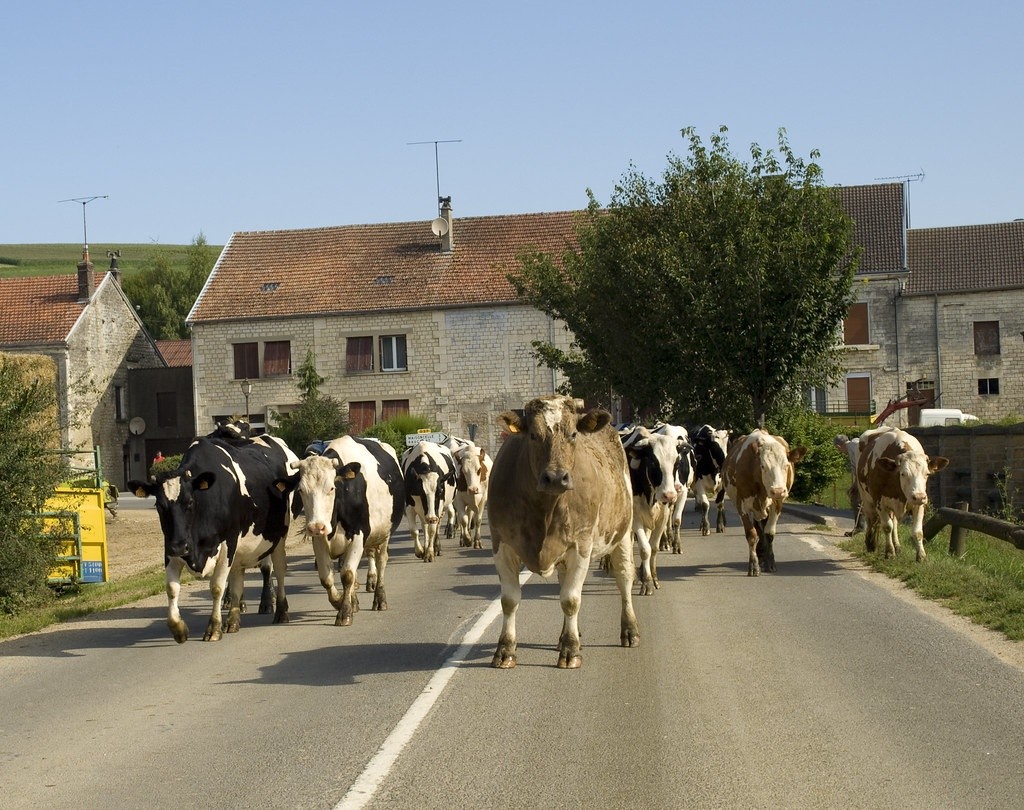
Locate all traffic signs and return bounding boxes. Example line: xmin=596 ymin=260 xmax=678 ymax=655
xmin=406 ymin=431 xmax=449 ymax=446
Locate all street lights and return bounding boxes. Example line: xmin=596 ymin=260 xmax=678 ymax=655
xmin=240 ymin=377 xmax=252 ymax=426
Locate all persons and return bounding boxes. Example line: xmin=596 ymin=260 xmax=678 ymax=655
xmin=153 ymin=451 xmax=165 ymax=464
xmin=834 ymin=435 xmax=866 ymax=536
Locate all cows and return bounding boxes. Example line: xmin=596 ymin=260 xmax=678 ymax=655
xmin=124 ymin=393 xmax=949 ymax=669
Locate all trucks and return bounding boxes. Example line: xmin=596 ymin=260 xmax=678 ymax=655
xmin=920 ymin=408 xmax=983 ymax=428
